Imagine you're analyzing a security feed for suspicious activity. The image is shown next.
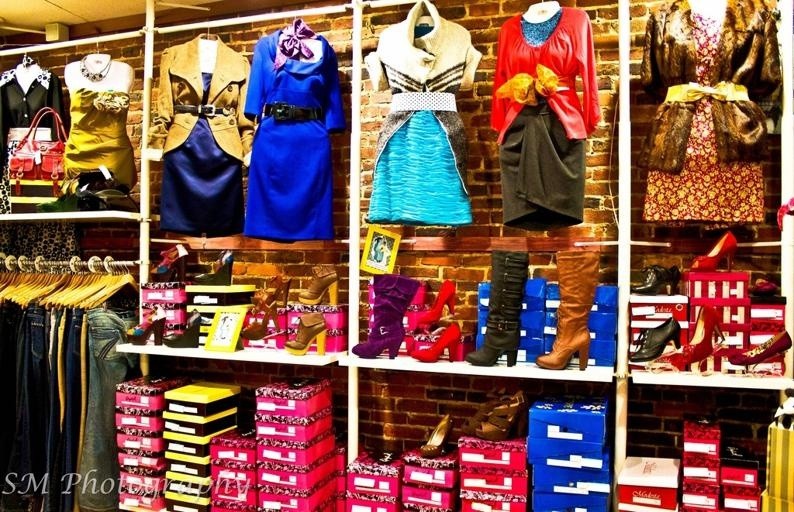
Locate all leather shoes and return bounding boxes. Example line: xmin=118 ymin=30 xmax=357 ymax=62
xmin=419 ymin=414 xmax=453 ymax=458
xmin=728 ymin=330 xmax=792 ymax=365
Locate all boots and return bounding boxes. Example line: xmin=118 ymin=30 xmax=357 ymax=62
xmin=352 ymin=273 xmax=420 ymax=360
xmin=535 ymin=249 xmax=600 ymax=370
xmin=464 ymin=249 xmax=529 ymax=366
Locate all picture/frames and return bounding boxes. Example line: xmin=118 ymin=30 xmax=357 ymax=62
xmin=357 ymin=226 xmax=401 ymax=276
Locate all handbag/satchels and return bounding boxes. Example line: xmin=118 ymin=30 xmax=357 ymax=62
xmin=9 ymin=106 xmax=68 ymax=180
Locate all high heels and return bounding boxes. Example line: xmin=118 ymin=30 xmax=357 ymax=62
xmin=630 ymin=317 xmax=681 ymax=362
xmin=632 ymin=265 xmax=681 ymax=296
xmin=682 ymin=305 xmax=725 ymax=364
xmin=125 ymin=304 xmax=201 ymax=348
xmin=690 ymin=231 xmax=738 ymax=272
xmin=411 ymin=280 xmax=461 ymax=362
xmin=239 ymin=264 xmax=338 ymax=355
xmin=150 ymin=244 xmax=233 ymax=285
xmin=461 ymin=385 xmax=527 ymax=440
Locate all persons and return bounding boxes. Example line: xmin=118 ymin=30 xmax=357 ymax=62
xmin=64 ymin=51 xmax=139 ymax=199
xmin=242 ymin=17 xmax=347 ymax=245
xmin=638 ymin=1 xmax=782 ymax=228
xmin=492 ymin=2 xmax=604 ymax=232
xmin=0 ymin=53 xmax=64 ymax=184
xmin=143 ymin=30 xmax=256 ymax=239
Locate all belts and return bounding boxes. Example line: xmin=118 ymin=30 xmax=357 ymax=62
xmin=265 ymin=101 xmax=322 ymax=123
xmin=174 ymin=104 xmax=224 ymax=118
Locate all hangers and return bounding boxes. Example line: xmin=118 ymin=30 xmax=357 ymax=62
xmin=0 ymin=252 xmax=139 ymax=311
